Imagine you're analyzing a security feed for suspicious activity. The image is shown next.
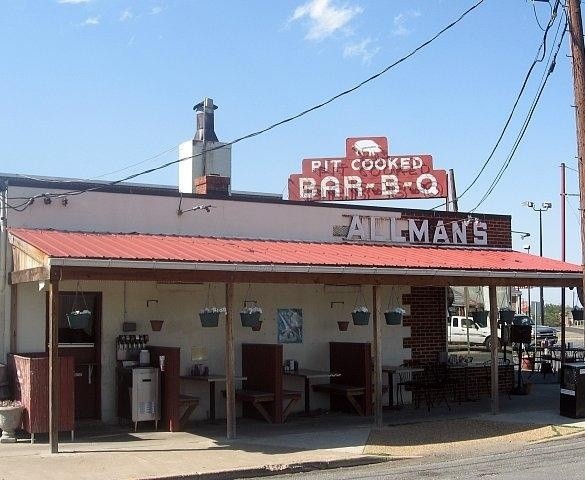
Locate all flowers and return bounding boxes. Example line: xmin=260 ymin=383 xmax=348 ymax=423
xmin=474 ymin=304 xmax=485 ymax=312
xmin=498 ymin=305 xmax=516 ymax=311
xmin=70 ymin=310 xmax=91 ymax=316
xmin=385 ymin=308 xmax=406 ymax=314
xmin=351 ymin=306 xmax=368 ymax=312
xmin=240 ymin=306 xmax=262 ymax=315
xmin=203 ymin=306 xmax=227 ymax=315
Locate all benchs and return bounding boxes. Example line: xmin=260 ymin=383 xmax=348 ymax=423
xmin=282 ymin=364 xmax=367 ymax=418
xmin=179 ymin=359 xmax=274 ymax=430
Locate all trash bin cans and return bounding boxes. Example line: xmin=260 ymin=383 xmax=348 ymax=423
xmin=560 ymin=361 xmax=585 ymax=419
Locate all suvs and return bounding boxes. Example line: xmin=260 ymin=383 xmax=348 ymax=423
xmin=512 ymin=313 xmax=558 ymax=350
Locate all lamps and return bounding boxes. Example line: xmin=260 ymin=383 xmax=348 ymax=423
xmin=509 ymin=324 xmax=534 ymax=395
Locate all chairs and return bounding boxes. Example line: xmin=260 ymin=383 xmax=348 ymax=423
xmin=383 ymin=362 xmax=452 ymax=412
xmin=513 ymin=342 xmax=585 ymax=381
xmin=476 ymin=358 xmax=513 ymax=401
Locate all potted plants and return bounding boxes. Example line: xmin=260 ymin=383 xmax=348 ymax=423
xmin=0 ymin=399 xmax=24 ymax=443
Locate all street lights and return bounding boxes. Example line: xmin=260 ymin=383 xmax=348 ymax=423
xmin=526 ymin=200 xmax=553 ymax=324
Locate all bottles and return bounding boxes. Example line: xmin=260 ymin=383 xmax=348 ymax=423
xmin=117 ymin=333 xmax=150 ymax=353
xmin=449 ymin=354 xmax=473 ymax=365
xmin=540 ymin=336 xmax=548 ymax=348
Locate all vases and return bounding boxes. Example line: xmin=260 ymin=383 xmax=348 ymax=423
xmin=198 ymin=314 xmax=219 ymax=327
xmin=352 ymin=312 xmax=371 ymax=326
xmin=384 ymin=313 xmax=402 ymax=325
xmin=240 ymin=313 xmax=261 ymax=328
xmin=473 ymin=311 xmax=514 ymax=323
xmin=65 ymin=314 xmax=94 ymax=329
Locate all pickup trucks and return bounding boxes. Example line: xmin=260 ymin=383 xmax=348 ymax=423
xmin=448 ymin=314 xmax=503 ymax=352
